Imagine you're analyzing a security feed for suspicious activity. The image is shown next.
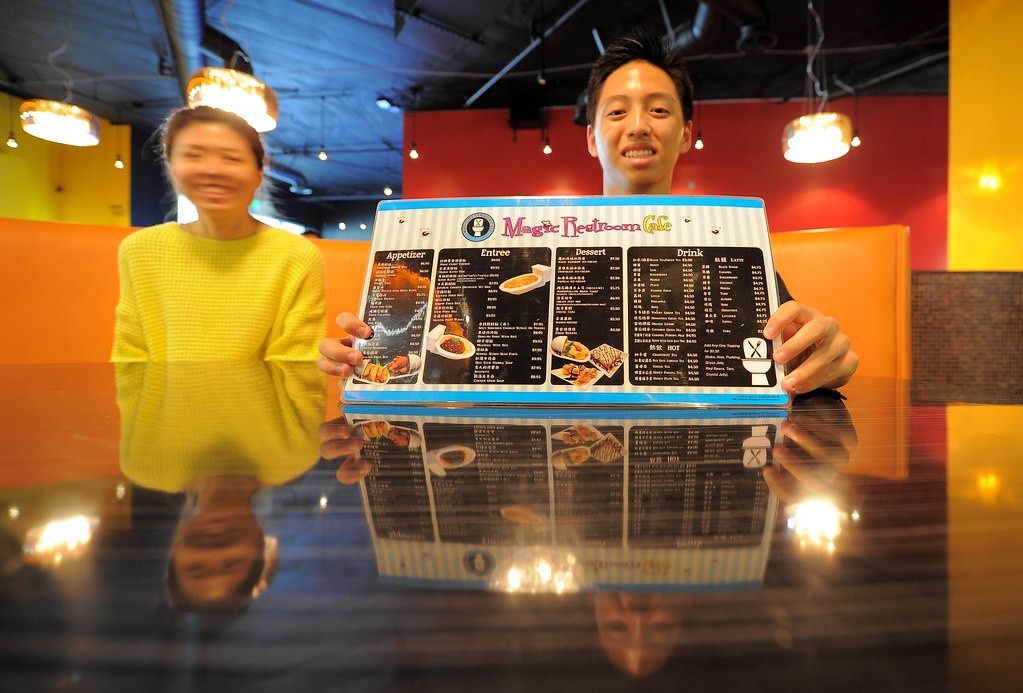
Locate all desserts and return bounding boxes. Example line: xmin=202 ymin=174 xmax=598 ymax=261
xmin=589 ymin=437 xmax=623 ymax=464
xmin=590 ymin=345 xmax=622 ymax=371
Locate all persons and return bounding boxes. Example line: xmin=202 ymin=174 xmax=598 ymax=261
xmin=110 ymin=107 xmax=326 ymax=361
xmin=114 ymin=362 xmax=328 ymax=621
xmin=321 ymin=404 xmax=859 ymax=675
xmin=319 ymin=29 xmax=860 ymax=393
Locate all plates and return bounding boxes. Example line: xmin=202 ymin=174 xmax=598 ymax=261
xmin=500 ymin=509 xmax=540 ymax=523
xmin=387 ymin=362 xmax=419 ymax=379
xmin=589 ymin=344 xmax=627 ymax=377
xmin=499 ymin=273 xmax=542 ymax=292
xmin=550 ymin=341 xmax=590 ymax=362
xmin=552 ymin=446 xmax=590 ymax=467
xmin=589 ymin=433 xmax=623 ymax=457
xmin=435 ymin=446 xmax=476 ymax=468
xmin=436 ymin=335 xmax=475 ymax=359
xmin=551 ymin=368 xmax=604 ymax=390
xmin=354 ymin=364 xmax=389 ymax=384
xmin=353 ymin=420 xmax=390 ymax=432
xmin=390 ymin=426 xmax=422 ymax=448
xmin=552 ymin=425 xmax=604 ymax=441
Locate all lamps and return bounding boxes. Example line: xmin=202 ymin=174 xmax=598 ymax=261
xmin=183 ymin=52 xmax=279 ymax=131
xmin=780 ymin=5 xmax=862 ymax=164
xmin=20 ymin=82 xmax=101 ymax=147
